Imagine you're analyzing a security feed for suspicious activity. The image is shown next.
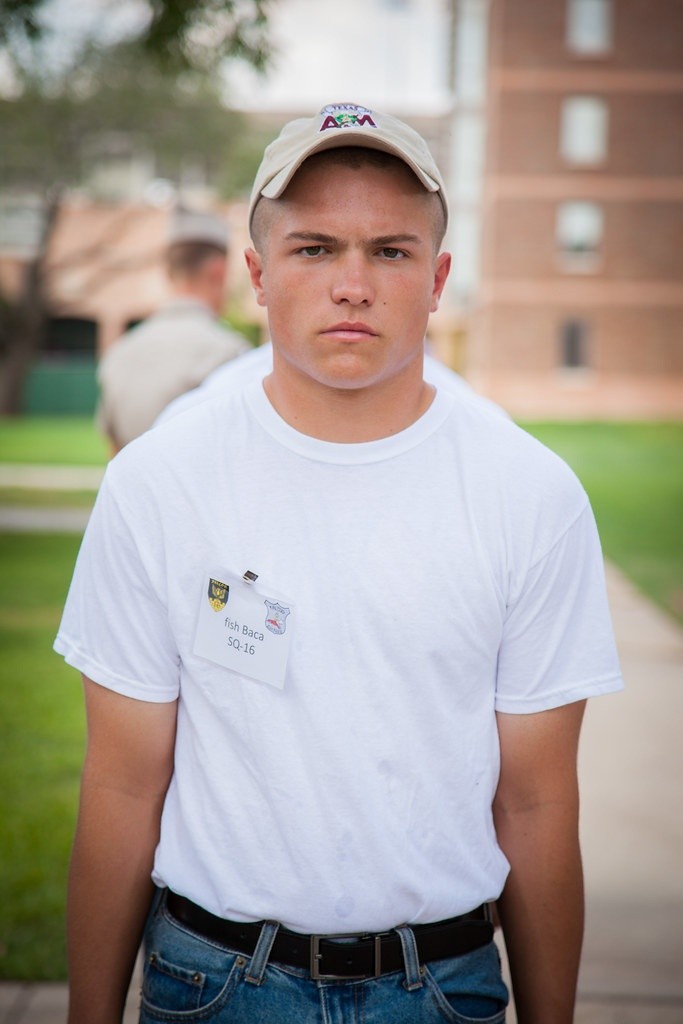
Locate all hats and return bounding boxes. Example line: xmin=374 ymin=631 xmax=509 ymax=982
xmin=245 ymin=103 xmax=449 ymax=247
xmin=165 ymin=219 xmax=229 ymax=255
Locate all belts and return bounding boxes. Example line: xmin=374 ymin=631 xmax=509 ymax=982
xmin=161 ymin=882 xmax=493 ymax=982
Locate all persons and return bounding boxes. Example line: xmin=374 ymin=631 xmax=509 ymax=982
xmin=89 ymin=225 xmax=254 ymax=458
xmin=53 ymin=99 xmax=624 ymax=1024
